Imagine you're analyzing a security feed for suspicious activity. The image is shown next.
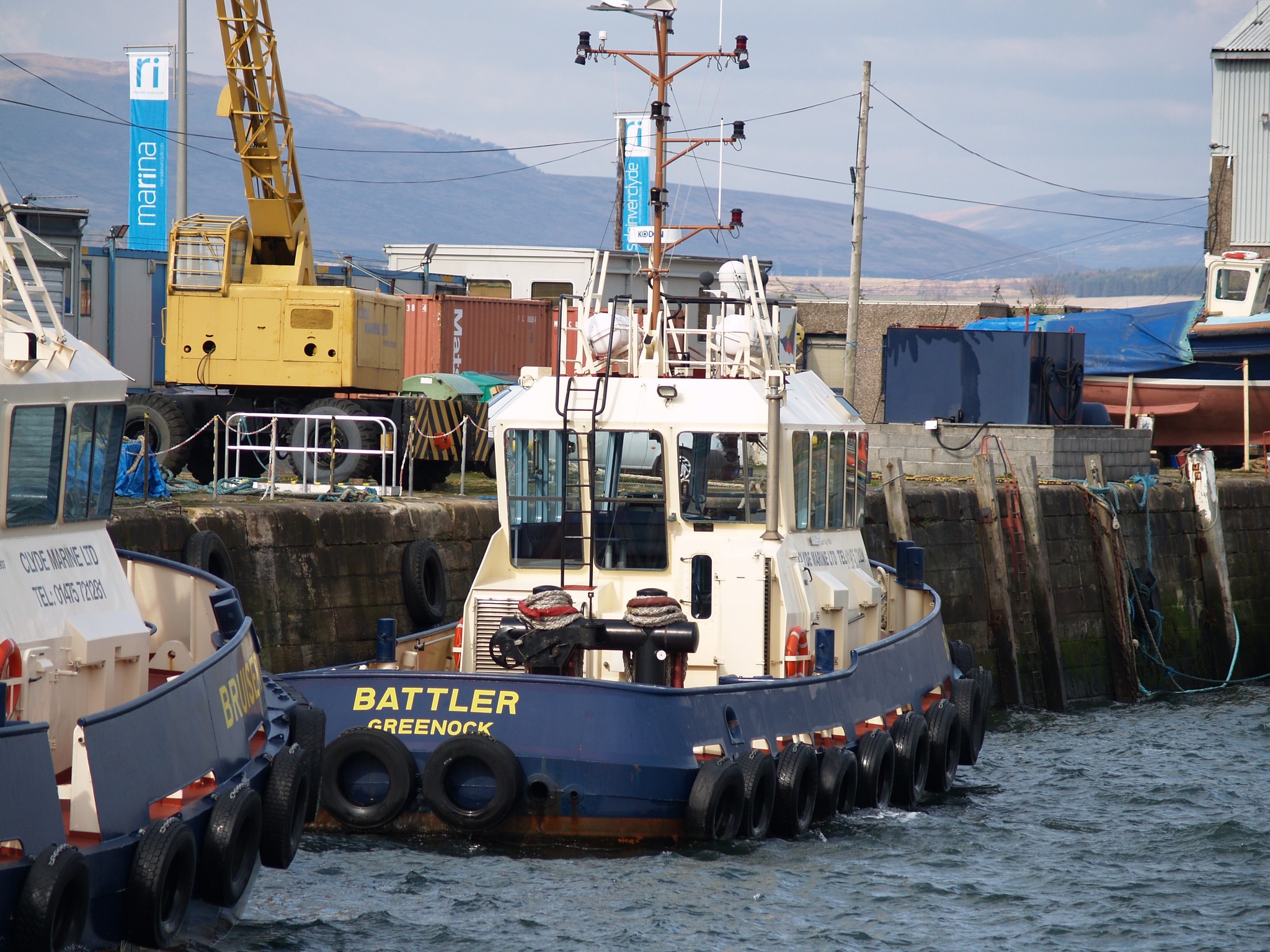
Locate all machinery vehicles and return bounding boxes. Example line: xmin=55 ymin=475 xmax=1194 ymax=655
xmin=122 ymin=0 xmax=495 ymax=491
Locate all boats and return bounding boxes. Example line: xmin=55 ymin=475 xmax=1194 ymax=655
xmin=272 ymin=0 xmax=985 ymax=853
xmin=964 ymin=251 xmax=1270 ymax=445
xmin=0 ymin=171 xmax=307 ymax=952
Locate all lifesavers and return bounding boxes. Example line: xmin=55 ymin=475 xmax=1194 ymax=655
xmin=951 ymin=678 xmax=983 ymax=767
xmin=127 ymin=815 xmax=197 ymax=950
xmin=421 ymin=731 xmax=522 ymax=832
xmin=964 ymin=666 xmax=992 ymax=750
xmin=817 ymin=746 xmax=859 ymax=820
xmin=183 ymin=530 xmax=237 ymax=586
xmin=289 ymin=706 xmax=327 ymax=824
xmin=1128 ymin=566 xmax=1163 ymax=633
xmin=735 ymin=749 xmax=777 ymax=841
xmin=0 ymin=638 xmax=22 ymax=722
xmin=321 ymin=725 xmax=419 ymax=831
xmin=687 ymin=756 xmax=745 ymax=843
xmin=453 ymin=616 xmax=463 ymax=670
xmin=892 ymin=712 xmax=931 ymax=806
xmin=776 ymin=742 xmax=818 ymax=838
xmin=948 ymin=641 xmax=974 ymax=675
xmin=1222 ymin=250 xmax=1258 ymax=260
xmin=786 ymin=626 xmax=809 ymax=678
xmin=925 ymin=700 xmax=961 ymax=793
xmin=15 ymin=841 xmax=92 ymax=952
xmin=401 ymin=539 xmax=449 ymax=622
xmin=855 ymin=729 xmax=896 ymax=809
xmin=201 ymin=783 xmax=263 ymax=910
xmin=259 ymin=746 xmax=313 ymax=869
